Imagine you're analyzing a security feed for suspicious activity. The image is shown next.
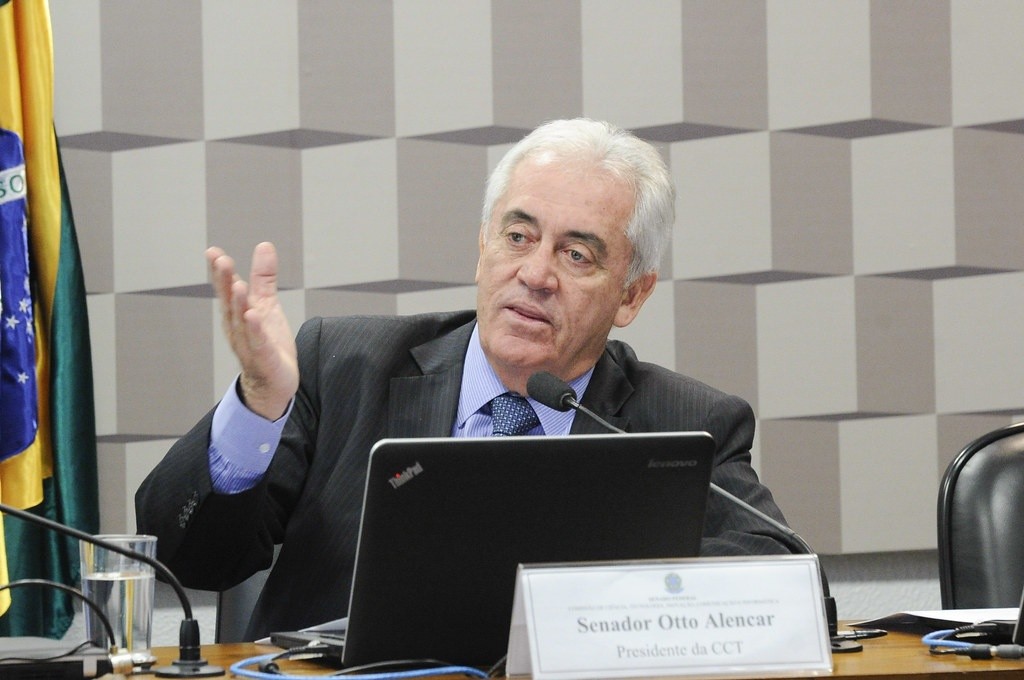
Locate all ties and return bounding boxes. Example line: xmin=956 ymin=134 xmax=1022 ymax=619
xmin=490 ymin=394 xmax=541 ymax=438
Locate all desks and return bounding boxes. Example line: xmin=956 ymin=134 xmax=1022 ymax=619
xmin=90 ymin=619 xmax=1024 ymax=680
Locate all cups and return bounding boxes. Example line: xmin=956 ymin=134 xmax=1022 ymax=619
xmin=79 ymin=534 xmax=158 ymax=671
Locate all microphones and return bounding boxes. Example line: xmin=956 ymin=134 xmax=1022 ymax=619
xmin=527 ymin=370 xmax=863 ymax=652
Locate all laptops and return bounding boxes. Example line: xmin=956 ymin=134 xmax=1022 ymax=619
xmin=913 ymin=588 xmax=1024 ymax=644
xmin=270 ymin=431 xmax=715 ymax=669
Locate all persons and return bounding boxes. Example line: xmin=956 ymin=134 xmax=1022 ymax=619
xmin=135 ymin=117 xmax=831 ymax=642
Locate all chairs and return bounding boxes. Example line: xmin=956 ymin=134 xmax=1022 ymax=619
xmin=936 ymin=421 xmax=1024 ymax=609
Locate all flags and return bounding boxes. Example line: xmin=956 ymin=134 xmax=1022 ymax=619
xmin=0 ymin=0 xmax=101 ymax=638
